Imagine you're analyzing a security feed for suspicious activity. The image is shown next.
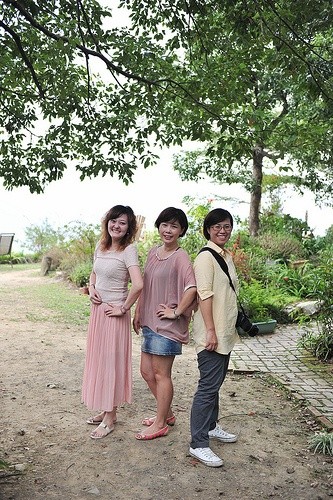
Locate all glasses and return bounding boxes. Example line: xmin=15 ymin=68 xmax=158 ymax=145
xmin=209 ymin=224 xmax=232 ymax=231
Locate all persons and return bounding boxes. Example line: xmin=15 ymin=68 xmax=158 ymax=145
xmin=189 ymin=209 xmax=241 ymax=467
xmin=80 ymin=205 xmax=144 ymax=439
xmin=133 ymin=207 xmax=196 ymax=440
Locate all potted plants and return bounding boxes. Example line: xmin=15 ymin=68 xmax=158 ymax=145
xmin=252 ymin=309 xmax=277 ymax=334
xmin=71 ymin=264 xmax=93 ymax=294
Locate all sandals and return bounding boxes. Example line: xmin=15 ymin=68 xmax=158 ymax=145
xmin=142 ymin=417 xmax=175 ymax=426
xmin=134 ymin=426 xmax=169 ymax=441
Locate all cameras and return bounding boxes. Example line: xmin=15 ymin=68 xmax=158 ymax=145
xmin=235 ymin=310 xmax=259 ymax=337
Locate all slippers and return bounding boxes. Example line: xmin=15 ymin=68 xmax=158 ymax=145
xmin=86 ymin=414 xmax=117 ymax=424
xmin=90 ymin=423 xmax=110 ymax=439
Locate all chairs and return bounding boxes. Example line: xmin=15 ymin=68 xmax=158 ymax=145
xmin=0 ymin=232 xmax=16 ymax=269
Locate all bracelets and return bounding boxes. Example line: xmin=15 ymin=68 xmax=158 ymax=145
xmin=174 ymin=307 xmax=179 ymax=320
xmin=119 ymin=305 xmax=128 ymax=314
xmin=90 ymin=283 xmax=95 ymax=287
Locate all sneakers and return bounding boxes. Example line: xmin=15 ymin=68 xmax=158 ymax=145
xmin=189 ymin=446 xmax=223 ymax=467
xmin=208 ymin=427 xmax=238 ymax=443
